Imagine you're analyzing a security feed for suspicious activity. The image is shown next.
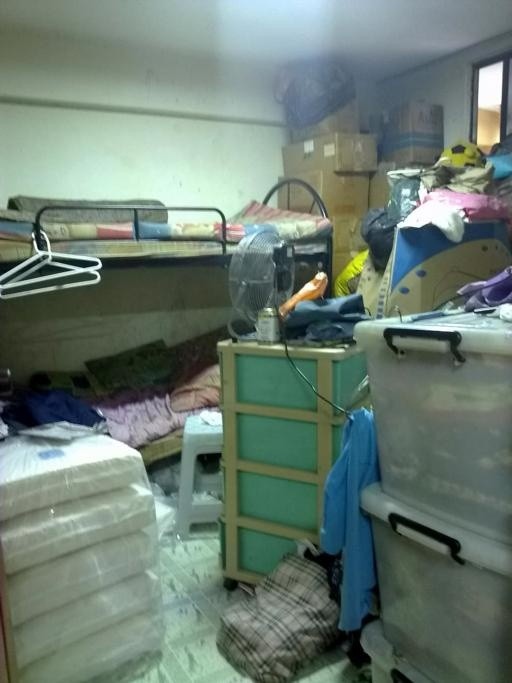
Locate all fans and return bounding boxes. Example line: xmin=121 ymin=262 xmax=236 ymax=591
xmin=224 ymin=223 xmax=298 ymax=333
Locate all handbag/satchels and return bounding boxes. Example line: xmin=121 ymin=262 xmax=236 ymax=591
xmin=216 ymin=539 xmax=341 ymax=683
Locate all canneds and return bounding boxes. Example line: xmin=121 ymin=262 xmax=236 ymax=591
xmin=257 ymin=308 xmax=280 ymax=345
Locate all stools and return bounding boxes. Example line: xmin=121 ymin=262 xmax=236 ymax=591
xmin=170 ymin=412 xmax=228 ymax=541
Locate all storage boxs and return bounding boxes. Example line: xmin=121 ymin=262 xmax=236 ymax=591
xmin=272 ymin=90 xmax=448 ymax=292
xmin=349 ymin=306 xmax=512 ymax=544
xmin=356 ymin=479 xmax=511 ymax=681
xmin=356 ymin=618 xmax=431 ymax=682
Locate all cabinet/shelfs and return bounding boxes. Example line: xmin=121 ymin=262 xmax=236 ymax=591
xmin=211 ymin=335 xmax=364 ymax=592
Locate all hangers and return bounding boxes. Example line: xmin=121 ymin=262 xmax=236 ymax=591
xmin=0 ymin=236 xmax=103 ymax=303
xmin=0 ymin=227 xmax=108 ymax=293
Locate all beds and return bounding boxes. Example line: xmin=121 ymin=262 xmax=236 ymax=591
xmin=1 ymin=171 xmax=334 ymax=481
